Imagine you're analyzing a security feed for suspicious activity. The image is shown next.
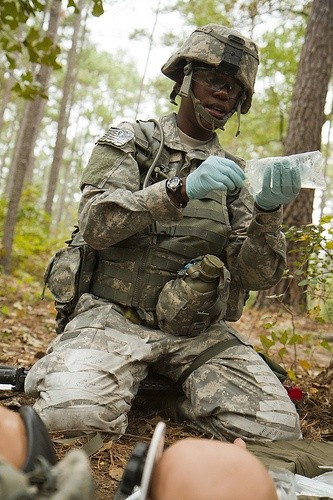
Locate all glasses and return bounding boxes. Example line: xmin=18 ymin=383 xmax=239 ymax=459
xmin=189 ymin=66 xmax=245 ymax=100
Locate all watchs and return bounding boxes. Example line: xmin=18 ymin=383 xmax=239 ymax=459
xmin=166 ymin=176 xmax=187 ymax=207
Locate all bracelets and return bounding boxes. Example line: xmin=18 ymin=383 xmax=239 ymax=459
xmin=253 ymin=200 xmax=281 ymax=213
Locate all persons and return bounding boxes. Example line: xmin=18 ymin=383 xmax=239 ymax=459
xmin=24 ymin=23 xmax=303 ymax=445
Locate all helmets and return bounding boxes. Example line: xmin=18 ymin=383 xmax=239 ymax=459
xmin=161 ymin=23 xmax=262 ymax=114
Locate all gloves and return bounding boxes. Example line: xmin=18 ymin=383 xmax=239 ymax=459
xmin=254 ymin=159 xmax=300 ymax=210
xmin=185 ymin=156 xmax=245 ymax=201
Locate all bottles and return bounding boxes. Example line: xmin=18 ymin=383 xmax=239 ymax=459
xmin=156 ymin=254 xmax=229 ymax=338
